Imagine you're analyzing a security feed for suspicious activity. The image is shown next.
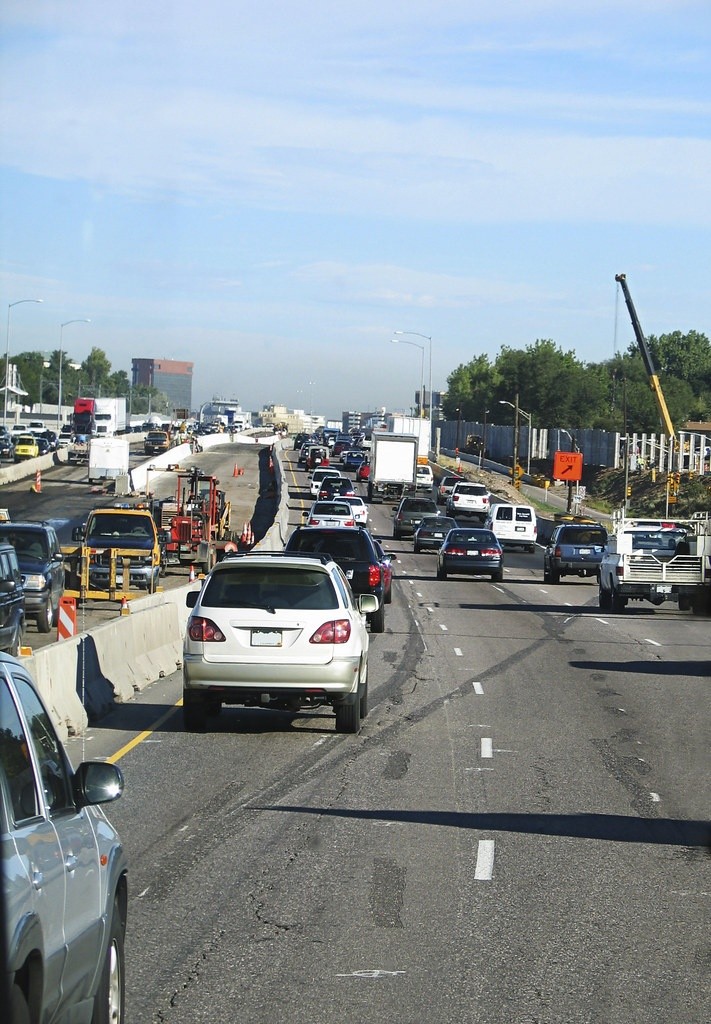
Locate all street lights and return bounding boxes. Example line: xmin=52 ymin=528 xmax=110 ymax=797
xmin=56 ymin=318 xmax=91 ymax=430
xmin=3 ymin=298 xmax=43 ymax=430
xmin=455 ymin=409 xmax=462 ymax=449
xmin=497 ymin=400 xmax=532 ymax=476
xmin=481 ymin=409 xmax=491 ymax=470
xmin=393 ymin=331 xmax=433 ymax=451
xmin=390 ymin=338 xmax=425 ymax=420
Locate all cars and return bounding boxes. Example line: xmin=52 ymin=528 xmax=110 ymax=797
xmin=0 ymin=398 xmax=245 ymax=465
xmin=695 ymin=446 xmax=710 ymax=461
xmin=434 ymin=528 xmax=505 ymax=582
xmin=294 ymin=426 xmax=492 ymax=604
xmin=181 ymin=550 xmax=381 ymax=734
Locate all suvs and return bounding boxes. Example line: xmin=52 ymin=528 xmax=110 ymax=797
xmin=283 ymin=525 xmax=391 ymax=634
xmin=0 ymin=541 xmax=28 ymax=658
xmin=0 ymin=646 xmax=132 ymax=1024
xmin=543 ymin=521 xmax=608 ymax=585
xmin=144 ymin=431 xmax=171 ymax=455
xmin=0 ymin=519 xmax=67 ymax=632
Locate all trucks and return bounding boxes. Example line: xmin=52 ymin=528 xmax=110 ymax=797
xmin=596 ymin=507 xmax=711 ymax=616
xmin=387 ymin=415 xmax=431 ymax=466
xmin=366 ymin=430 xmax=420 ymax=503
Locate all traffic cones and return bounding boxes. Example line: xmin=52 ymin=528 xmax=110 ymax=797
xmin=457 ymin=462 xmax=463 ymax=473
xmin=189 ymin=566 xmax=195 ymax=583
xmin=240 ymin=521 xmax=255 ymax=549
xmin=121 ymin=597 xmax=127 ymax=610
xmin=232 ymin=463 xmax=238 ymax=478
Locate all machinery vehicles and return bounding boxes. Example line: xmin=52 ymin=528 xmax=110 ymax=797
xmin=614 ymin=271 xmax=700 ymax=474
xmin=155 ymin=469 xmax=238 ymax=578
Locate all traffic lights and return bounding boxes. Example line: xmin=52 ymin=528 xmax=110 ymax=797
xmin=667 ymin=473 xmax=679 ymax=496
xmin=689 ymin=469 xmax=694 ymax=480
xmin=676 ymin=474 xmax=680 ymax=484
xmin=627 ymin=486 xmax=632 ymax=496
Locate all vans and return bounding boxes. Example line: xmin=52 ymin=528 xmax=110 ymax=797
xmin=71 ymin=503 xmax=172 ymax=593
xmin=484 ymin=503 xmax=538 ymax=553
